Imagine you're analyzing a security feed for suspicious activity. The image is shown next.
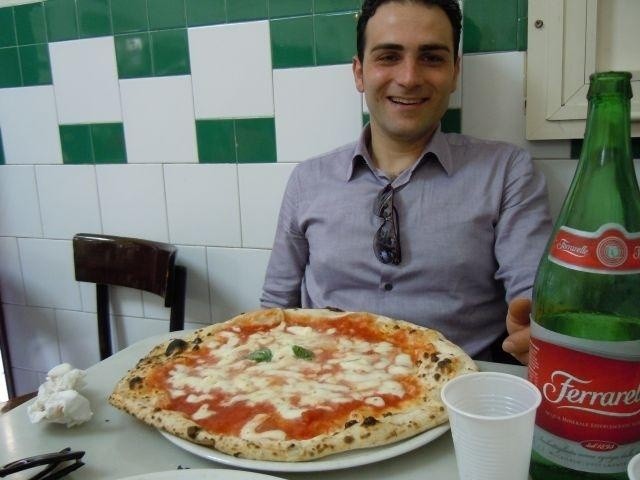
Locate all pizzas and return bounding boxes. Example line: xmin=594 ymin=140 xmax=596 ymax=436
xmin=108 ymin=306 xmax=479 ymax=461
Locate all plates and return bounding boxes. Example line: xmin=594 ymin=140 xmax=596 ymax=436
xmin=157 ymin=420 xmax=451 ymax=473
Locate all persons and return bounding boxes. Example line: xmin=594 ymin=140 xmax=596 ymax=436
xmin=260 ymin=1 xmax=558 ymax=363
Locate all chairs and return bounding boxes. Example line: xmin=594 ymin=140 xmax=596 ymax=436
xmin=1 ymin=232 xmax=187 ymax=421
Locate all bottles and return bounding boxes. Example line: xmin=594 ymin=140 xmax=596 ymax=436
xmin=529 ymin=72 xmax=639 ymax=479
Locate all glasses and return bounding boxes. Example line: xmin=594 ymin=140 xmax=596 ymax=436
xmin=372 ymin=184 xmax=402 ymax=265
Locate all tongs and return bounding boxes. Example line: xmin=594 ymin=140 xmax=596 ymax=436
xmin=0 ymin=448 xmax=86 ymax=480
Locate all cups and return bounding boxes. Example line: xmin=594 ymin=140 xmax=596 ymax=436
xmin=440 ymin=372 xmax=542 ymax=479
